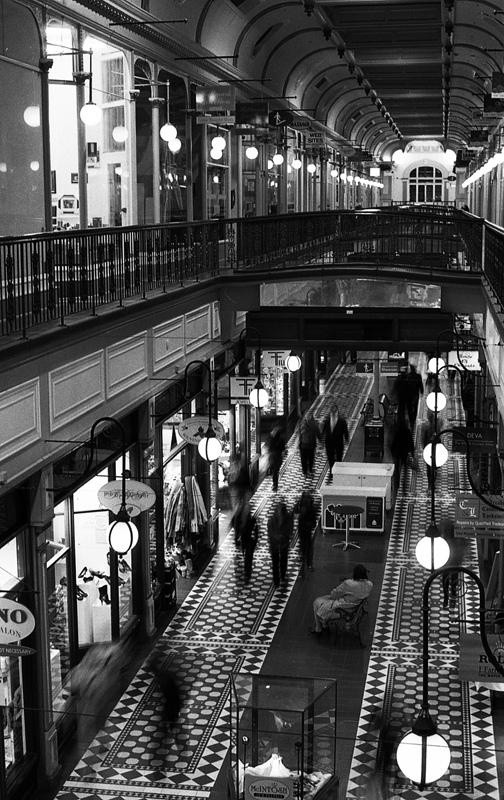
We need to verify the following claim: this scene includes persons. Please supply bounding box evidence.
[232,502,263,583]
[388,366,409,424]
[310,562,374,635]
[421,410,444,490]
[325,402,349,475]
[390,417,416,494]
[298,410,323,477]
[299,489,320,578]
[409,362,425,426]
[266,422,287,494]
[268,503,294,587]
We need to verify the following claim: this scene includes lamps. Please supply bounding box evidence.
[45,43,259,162]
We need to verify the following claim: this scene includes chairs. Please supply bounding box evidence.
[325,597,370,649]
[378,393,397,424]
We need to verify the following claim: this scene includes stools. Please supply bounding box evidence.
[330,505,364,551]
